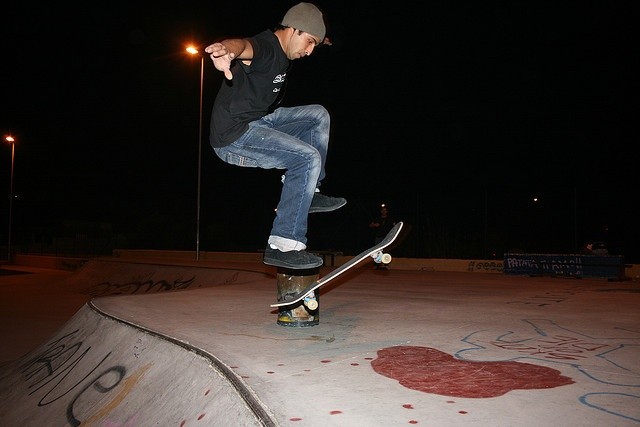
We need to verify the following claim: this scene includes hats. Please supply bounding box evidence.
[281,2,326,45]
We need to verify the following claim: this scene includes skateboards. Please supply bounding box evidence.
[271,222,404,310]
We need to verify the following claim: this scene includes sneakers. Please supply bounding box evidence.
[264,245,324,269]
[308,192,346,213]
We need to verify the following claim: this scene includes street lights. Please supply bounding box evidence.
[5,134,15,258]
[186,45,203,260]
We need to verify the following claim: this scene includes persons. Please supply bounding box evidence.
[204,3,347,269]
